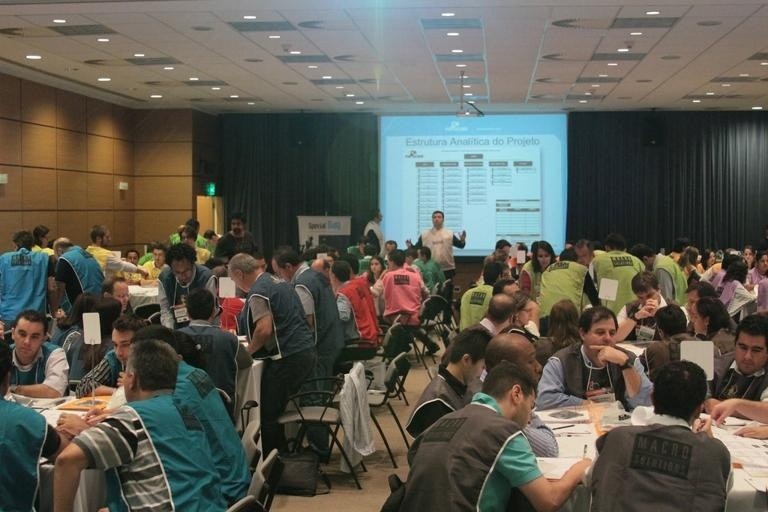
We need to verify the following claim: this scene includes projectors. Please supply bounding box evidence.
[456,112,480,118]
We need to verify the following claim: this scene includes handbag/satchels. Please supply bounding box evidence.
[261,437,322,499]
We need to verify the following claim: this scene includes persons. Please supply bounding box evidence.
[211,211,259,267]
[405,210,468,326]
[362,207,384,253]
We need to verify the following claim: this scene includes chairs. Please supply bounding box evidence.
[1,225,768,512]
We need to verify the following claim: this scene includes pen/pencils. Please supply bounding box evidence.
[552,425,574,430]
[567,435,583,437]
[583,445,587,459]
